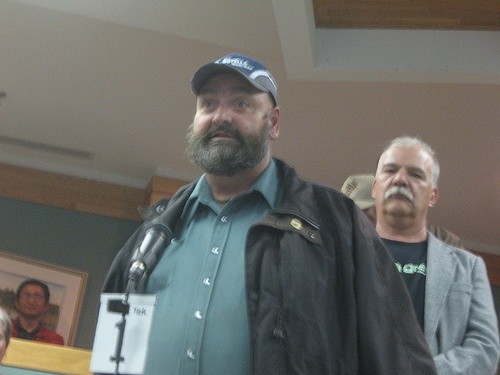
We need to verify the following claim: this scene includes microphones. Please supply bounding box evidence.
[128,219,173,281]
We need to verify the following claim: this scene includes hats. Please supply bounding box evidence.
[190,54,279,107]
[338,175,378,210]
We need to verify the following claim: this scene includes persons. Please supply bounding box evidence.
[0,309,13,364]
[98,54,437,375]
[339,138,500,375]
[9,278,65,346]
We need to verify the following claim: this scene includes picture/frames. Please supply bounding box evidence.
[0,252,89,361]
